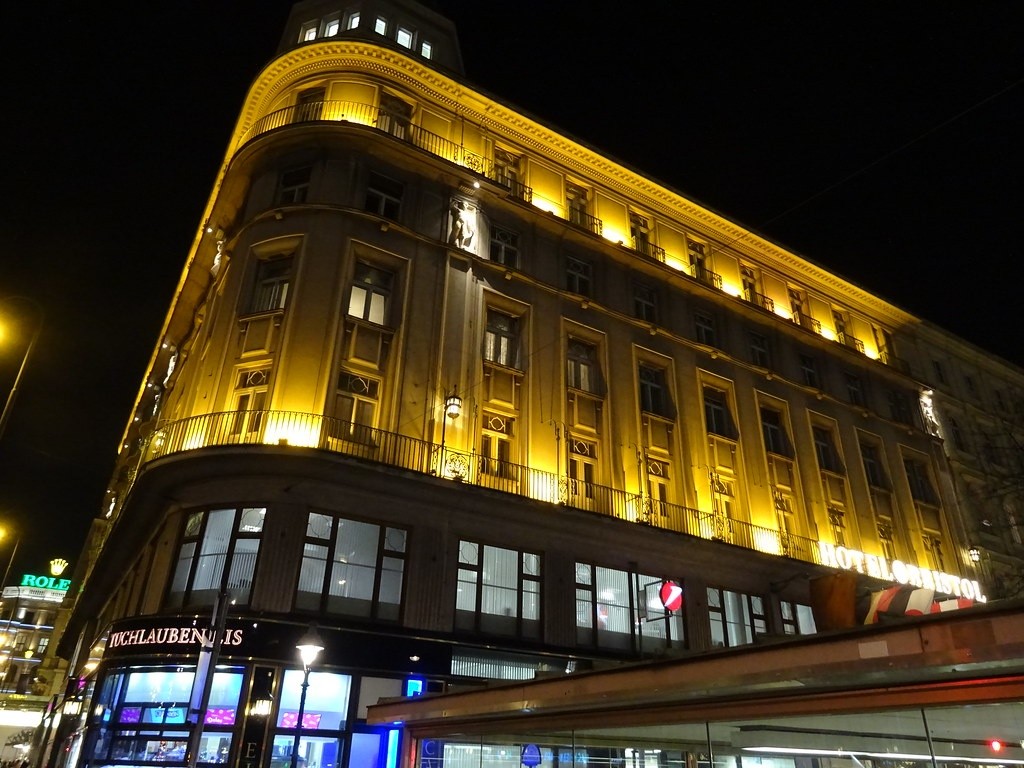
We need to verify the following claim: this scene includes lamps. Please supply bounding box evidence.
[446,385,462,420]
[968,545,980,566]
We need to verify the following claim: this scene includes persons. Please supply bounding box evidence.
[0,758,30,768]
[211,241,223,275]
[32,675,47,695]
[921,390,941,438]
[451,199,476,249]
[164,355,176,388]
[107,498,116,519]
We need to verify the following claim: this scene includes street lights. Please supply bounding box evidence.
[289,624,325,768]
